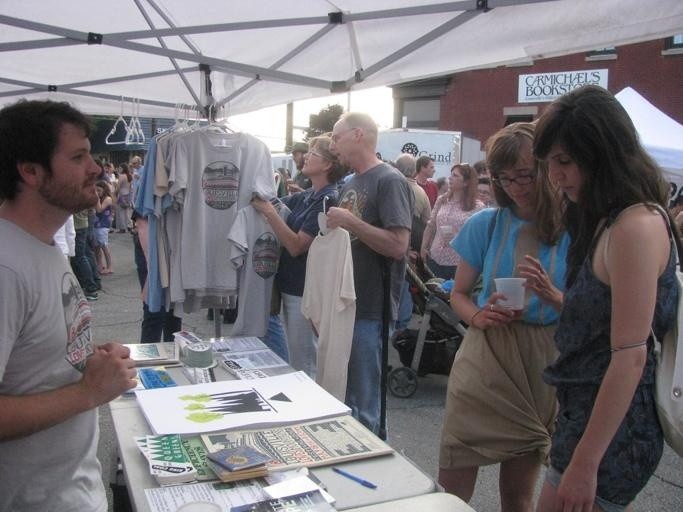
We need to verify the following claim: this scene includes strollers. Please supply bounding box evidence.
[380,259,470,397]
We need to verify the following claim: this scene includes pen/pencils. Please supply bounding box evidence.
[330,465,378,492]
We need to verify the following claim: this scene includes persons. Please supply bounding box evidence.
[94,160,114,185]
[128,155,146,226]
[89,181,114,275]
[476,176,493,203]
[72,207,105,301]
[531,84,682,507]
[285,180,307,195]
[416,156,438,206]
[439,124,567,508]
[277,142,309,190]
[326,111,413,441]
[53,211,76,262]
[394,151,431,305]
[474,163,494,182]
[113,163,132,232]
[420,162,486,278]
[132,211,182,344]
[2,98,136,508]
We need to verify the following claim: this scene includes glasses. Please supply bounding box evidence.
[310,149,329,161]
[330,127,357,145]
[492,173,537,188]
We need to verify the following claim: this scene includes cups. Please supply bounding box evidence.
[494,278,527,311]
[439,225,452,240]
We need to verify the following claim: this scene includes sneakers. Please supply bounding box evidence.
[85,292,98,301]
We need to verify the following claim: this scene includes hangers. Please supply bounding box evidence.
[105,96,135,145]
[318,195,330,235]
[198,103,222,339]
[127,97,146,145]
[268,197,283,208]
[124,97,140,145]
[152,103,239,146]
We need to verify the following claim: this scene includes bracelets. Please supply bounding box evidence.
[470,309,481,325]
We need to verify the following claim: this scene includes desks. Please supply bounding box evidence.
[336,492,478,512]
[109,335,435,512]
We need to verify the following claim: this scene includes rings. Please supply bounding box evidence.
[488,304,498,313]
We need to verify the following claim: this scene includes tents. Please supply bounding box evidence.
[0,1,645,124]
